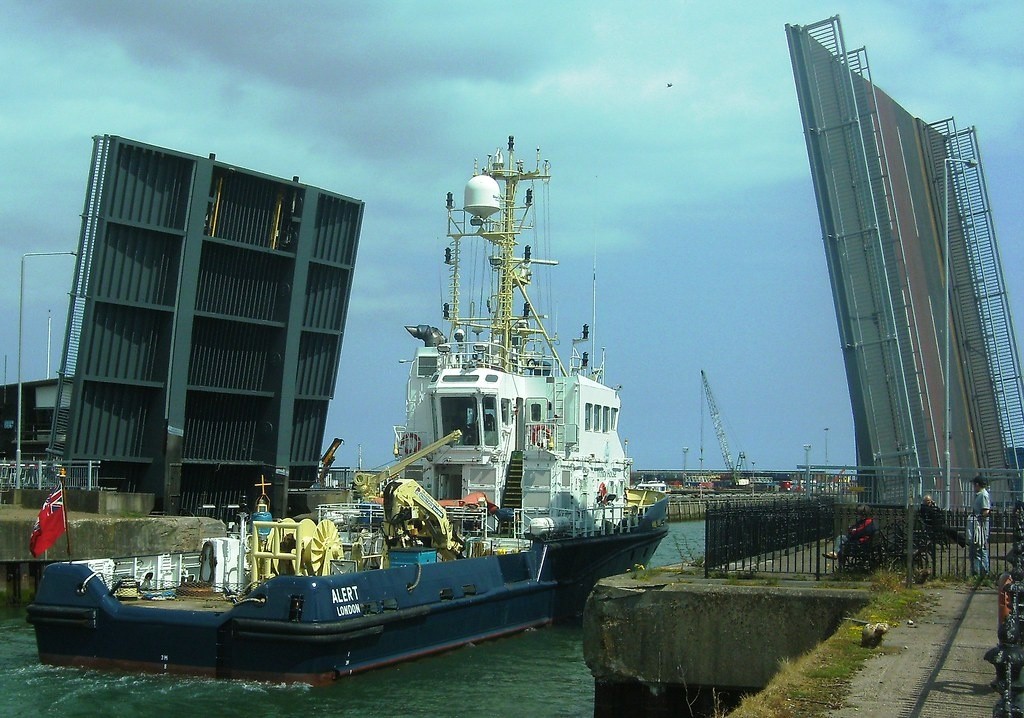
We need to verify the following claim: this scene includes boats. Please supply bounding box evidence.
[25,135,670,687]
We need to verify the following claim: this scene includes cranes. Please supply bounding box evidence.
[699,370,736,475]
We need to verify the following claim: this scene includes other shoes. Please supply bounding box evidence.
[821,551,838,561]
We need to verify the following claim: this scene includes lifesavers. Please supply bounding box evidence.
[400,433,422,454]
[531,425,551,449]
[599,482,607,504]
[997,576,1013,625]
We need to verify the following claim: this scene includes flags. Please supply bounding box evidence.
[29,483,66,559]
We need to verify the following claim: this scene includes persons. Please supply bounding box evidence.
[822,505,876,575]
[921,494,970,549]
[966,475,991,575]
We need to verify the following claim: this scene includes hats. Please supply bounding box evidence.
[969,475,987,485]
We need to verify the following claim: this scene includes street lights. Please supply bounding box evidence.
[46,309,52,379]
[943,158,979,512]
[682,447,689,473]
[751,461,756,498]
[803,443,812,497]
[824,428,829,465]
[15,250,77,488]
